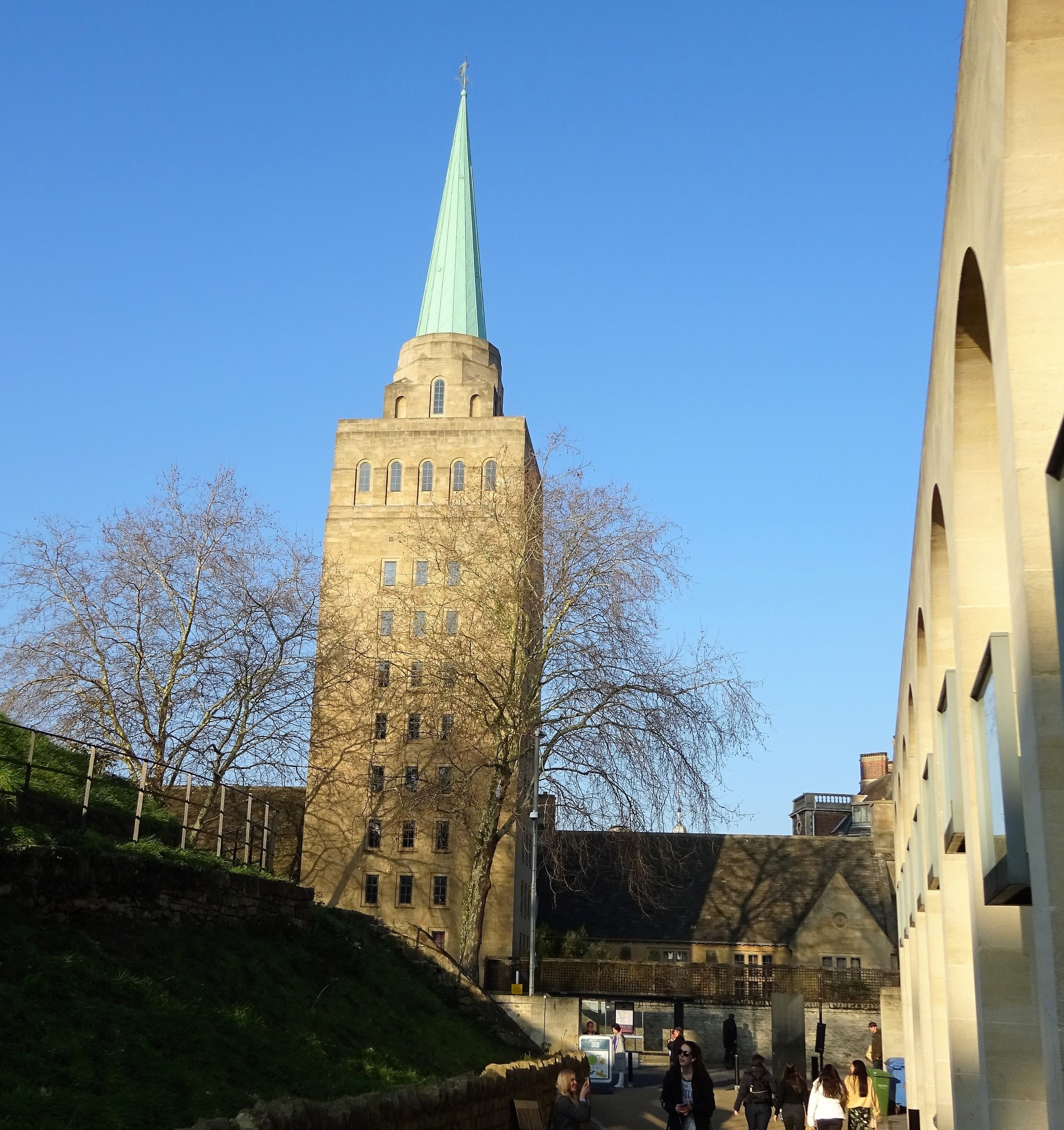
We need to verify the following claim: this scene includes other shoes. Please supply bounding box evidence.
[614,1085,624,1088]
[723,1064,735,1069]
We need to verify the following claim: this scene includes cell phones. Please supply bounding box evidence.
[585,1077,589,1087]
[679,1103,687,1107]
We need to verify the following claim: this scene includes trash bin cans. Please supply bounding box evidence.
[883,1058,906,1115]
[866,1068,892,1116]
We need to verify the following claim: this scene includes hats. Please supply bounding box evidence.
[868,1022,877,1027]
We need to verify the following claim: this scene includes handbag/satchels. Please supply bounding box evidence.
[865,1045,874,1064]
[612,1052,626,1070]
[869,1118,877,1129]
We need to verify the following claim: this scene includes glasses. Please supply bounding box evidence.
[677,1048,691,1057]
[868,1026,872,1028]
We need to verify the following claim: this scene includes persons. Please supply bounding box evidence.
[611,1023,626,1088]
[733,1054,778,1130]
[774,1062,809,1130]
[722,1013,737,1069]
[667,1027,686,1069]
[868,1022,883,1070]
[844,1060,880,1130]
[551,1070,590,1130]
[660,1041,716,1130]
[807,1064,850,1130]
[582,1021,600,1035]
